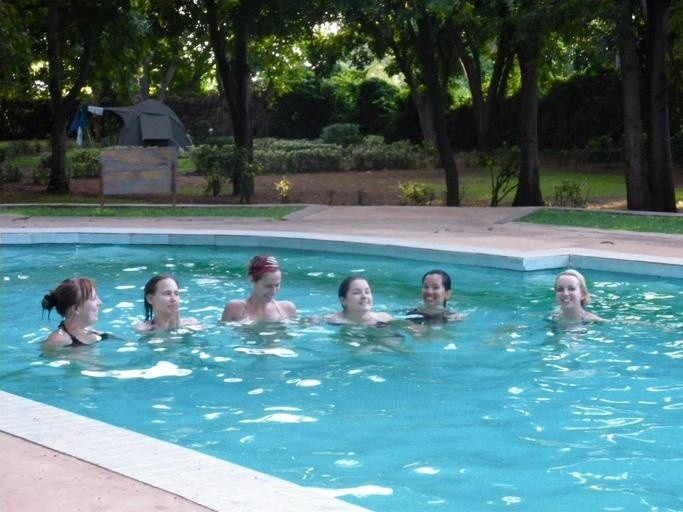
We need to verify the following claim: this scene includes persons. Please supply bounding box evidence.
[548,269,607,322]
[404,269,462,324]
[221,255,296,322]
[41,277,108,347]
[325,276,395,326]
[132,276,199,332]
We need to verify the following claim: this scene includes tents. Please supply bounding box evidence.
[103,98,186,148]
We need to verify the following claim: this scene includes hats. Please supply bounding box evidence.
[249,256,281,276]
